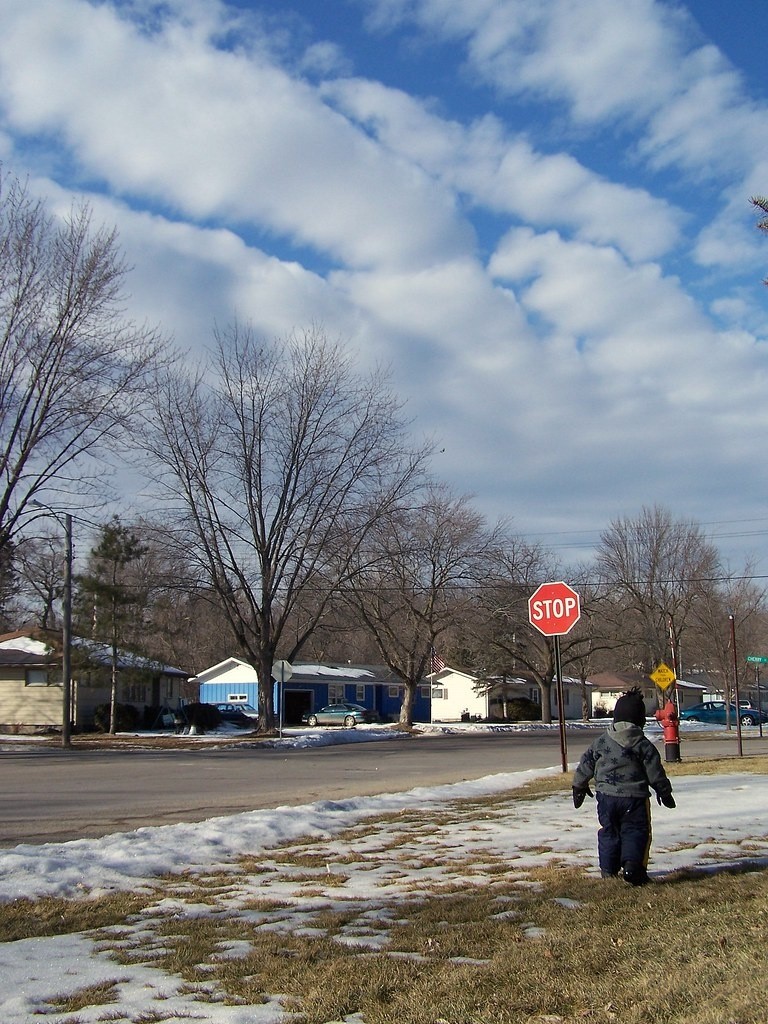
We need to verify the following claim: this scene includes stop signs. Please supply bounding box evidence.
[526,579,581,636]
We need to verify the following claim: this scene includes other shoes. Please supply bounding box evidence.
[623,861,650,886]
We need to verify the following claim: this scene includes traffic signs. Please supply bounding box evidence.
[746,656,768,664]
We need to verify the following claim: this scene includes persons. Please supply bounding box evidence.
[572,686,676,886]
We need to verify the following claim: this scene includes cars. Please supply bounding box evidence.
[212,703,277,724]
[302,705,378,726]
[679,700,766,726]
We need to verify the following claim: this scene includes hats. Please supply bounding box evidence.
[614,686,647,727]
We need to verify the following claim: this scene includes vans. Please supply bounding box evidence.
[734,699,757,710]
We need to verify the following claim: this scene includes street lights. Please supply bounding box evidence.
[27,499,71,748]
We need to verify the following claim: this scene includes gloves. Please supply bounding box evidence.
[657,793,675,808]
[572,786,593,808]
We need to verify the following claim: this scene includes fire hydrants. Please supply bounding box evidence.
[655,700,683,762]
